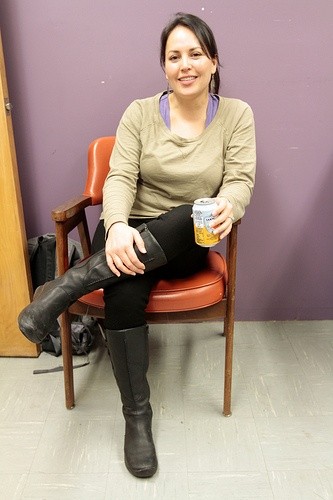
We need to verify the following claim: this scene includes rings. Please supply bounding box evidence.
[228,216,235,221]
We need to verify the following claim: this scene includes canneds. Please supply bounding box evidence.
[192,198,221,247]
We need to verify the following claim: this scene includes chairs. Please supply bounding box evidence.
[52,136,242,418]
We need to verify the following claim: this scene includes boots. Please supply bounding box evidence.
[104,323,158,479]
[17,223,168,345]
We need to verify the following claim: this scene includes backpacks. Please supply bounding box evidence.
[27,232,101,357]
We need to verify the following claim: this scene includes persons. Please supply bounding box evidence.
[18,12,256,479]
